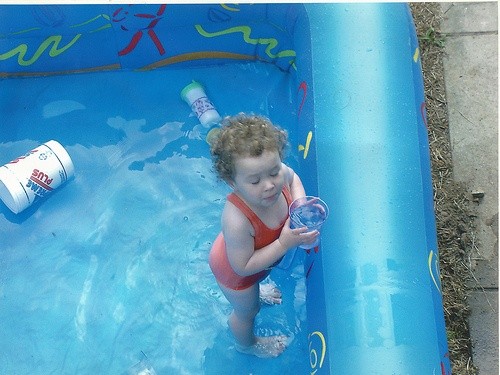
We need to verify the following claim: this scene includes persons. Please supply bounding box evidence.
[207,111,325,346]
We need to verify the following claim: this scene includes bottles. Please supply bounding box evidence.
[179,80,222,128]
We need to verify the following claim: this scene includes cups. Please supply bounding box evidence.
[288,196,329,250]
